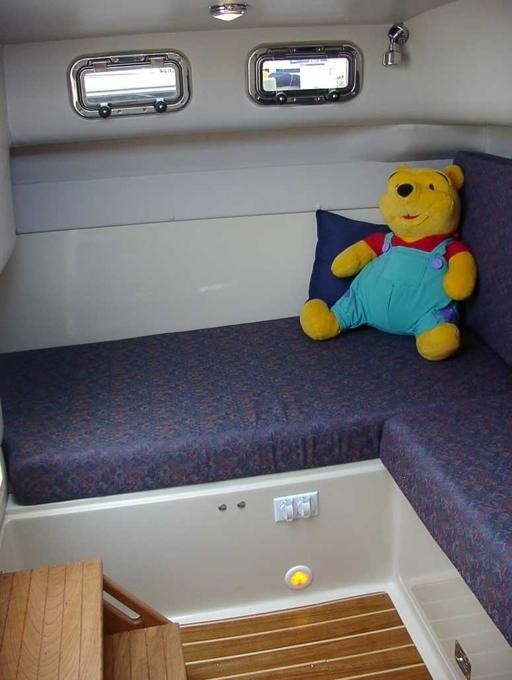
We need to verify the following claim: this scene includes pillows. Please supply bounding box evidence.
[308,209,390,311]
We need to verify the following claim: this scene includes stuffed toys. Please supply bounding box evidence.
[300,160,478,362]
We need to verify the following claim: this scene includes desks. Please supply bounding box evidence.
[0,557,189,680]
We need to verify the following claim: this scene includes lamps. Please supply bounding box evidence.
[210,3,247,21]
[382,22,409,67]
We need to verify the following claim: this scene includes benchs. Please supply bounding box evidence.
[1,150,512,680]
